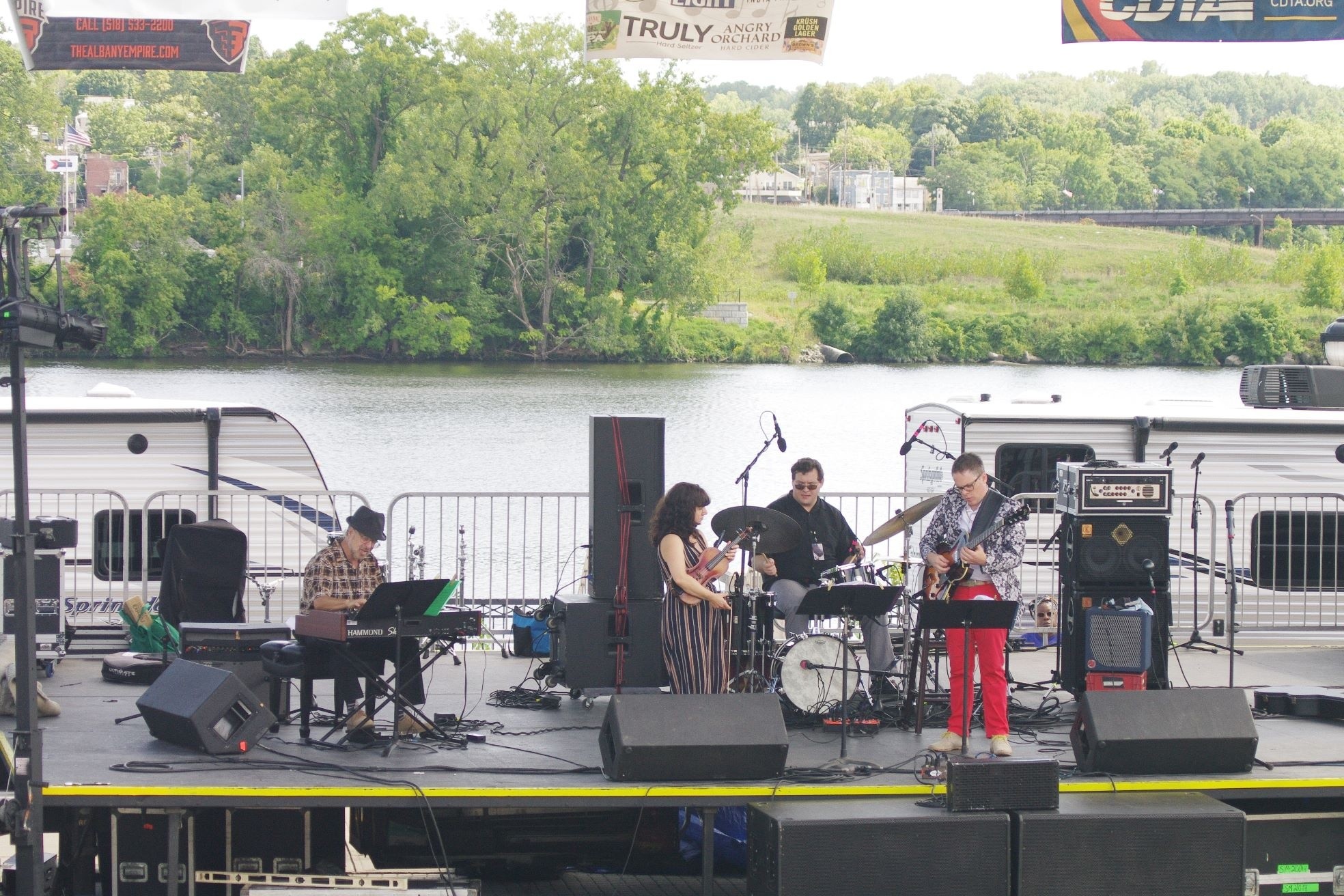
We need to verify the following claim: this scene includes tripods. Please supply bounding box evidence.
[346,579,450,757]
[974,525,1063,701]
[795,516,948,769]
[727,523,796,713]
[286,694,348,724]
[1169,465,1244,655]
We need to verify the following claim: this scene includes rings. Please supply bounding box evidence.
[937,570,939,572]
[964,561,968,564]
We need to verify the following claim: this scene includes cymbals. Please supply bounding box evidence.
[859,494,945,547]
[879,557,924,564]
[709,504,804,556]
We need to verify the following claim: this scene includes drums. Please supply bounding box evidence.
[717,591,778,656]
[819,561,884,618]
[769,632,861,717]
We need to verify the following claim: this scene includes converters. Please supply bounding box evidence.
[467,733,486,743]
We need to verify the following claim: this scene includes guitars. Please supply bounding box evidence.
[666,524,753,607]
[922,504,1032,600]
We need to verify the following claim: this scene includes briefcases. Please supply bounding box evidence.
[0,515,78,549]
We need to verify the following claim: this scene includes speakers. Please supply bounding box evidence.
[747,791,1248,896]
[179,621,292,722]
[588,415,665,602]
[946,757,1060,812]
[1069,688,1258,775]
[136,657,280,755]
[598,694,790,783]
[1060,512,1171,588]
[550,594,670,689]
[1062,585,1169,696]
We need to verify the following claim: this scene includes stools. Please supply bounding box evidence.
[258,637,392,746]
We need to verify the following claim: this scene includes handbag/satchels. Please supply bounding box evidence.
[120,603,180,653]
[508,606,550,657]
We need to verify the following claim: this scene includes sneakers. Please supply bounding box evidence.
[927,731,970,751]
[989,734,1012,756]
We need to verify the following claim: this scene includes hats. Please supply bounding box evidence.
[346,506,388,541]
[1028,596,1058,621]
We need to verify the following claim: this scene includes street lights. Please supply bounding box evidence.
[1251,214,1263,249]
[1246,185,1253,216]
[1153,188,1163,230]
[1015,212,1024,222]
[966,189,975,217]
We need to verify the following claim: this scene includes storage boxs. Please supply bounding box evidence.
[122,596,154,629]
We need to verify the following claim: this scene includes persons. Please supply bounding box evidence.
[747,457,908,692]
[299,506,432,733]
[919,452,1025,756]
[1020,595,1062,648]
[649,482,739,695]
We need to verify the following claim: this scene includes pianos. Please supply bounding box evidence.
[294,606,483,760]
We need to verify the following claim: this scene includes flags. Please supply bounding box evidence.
[66,122,92,146]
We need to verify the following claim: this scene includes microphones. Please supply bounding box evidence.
[1083,448,1094,462]
[1159,442,1178,459]
[900,422,926,455]
[806,662,815,670]
[1142,559,1156,591]
[1225,500,1234,543]
[1191,452,1206,469]
[773,415,786,452]
[849,538,857,556]
[264,677,291,683]
[735,573,740,588]
[875,565,893,573]
[715,529,726,548]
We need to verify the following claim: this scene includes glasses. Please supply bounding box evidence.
[794,480,820,490]
[1037,611,1054,618]
[358,531,380,548]
[953,474,981,494]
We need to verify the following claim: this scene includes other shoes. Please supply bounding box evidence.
[346,713,375,730]
[398,713,437,734]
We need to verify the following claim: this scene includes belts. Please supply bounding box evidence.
[959,580,993,587]
